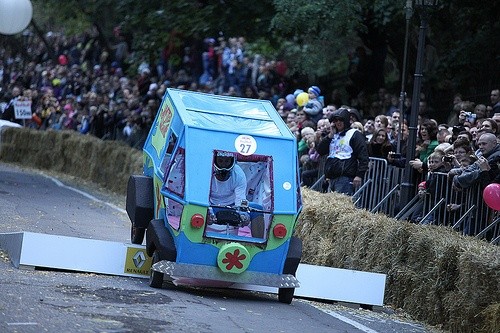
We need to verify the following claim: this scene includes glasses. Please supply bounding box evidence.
[334,118,344,122]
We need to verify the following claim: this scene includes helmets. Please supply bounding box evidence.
[214,150,236,173]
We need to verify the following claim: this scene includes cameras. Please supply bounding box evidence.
[320,127,330,137]
[453,126,463,133]
[464,112,471,118]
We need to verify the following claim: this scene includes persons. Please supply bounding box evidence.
[420,150,449,225]
[208,149,266,239]
[445,156,478,236]
[408,181,427,225]
[452,133,500,241]
[316,109,370,195]
[0,22,500,185]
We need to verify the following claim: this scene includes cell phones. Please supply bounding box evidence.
[391,153,401,159]
[444,157,452,162]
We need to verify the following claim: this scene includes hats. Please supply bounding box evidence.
[64,104,72,110]
[418,180,427,189]
[294,89,304,97]
[308,86,321,96]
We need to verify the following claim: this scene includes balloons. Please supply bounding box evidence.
[0,0,34,36]
[483,182,500,212]
